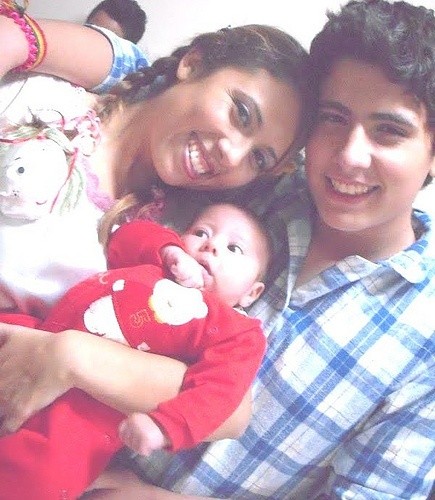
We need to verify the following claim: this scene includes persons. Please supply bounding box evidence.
[0,0,435,500]
[0,25,317,433]
[0,200,274,500]
[85,0,147,45]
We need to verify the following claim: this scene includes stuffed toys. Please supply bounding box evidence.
[1,106,103,231]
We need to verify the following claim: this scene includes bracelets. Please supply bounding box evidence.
[1,0,47,72]
[0,5,39,74]
